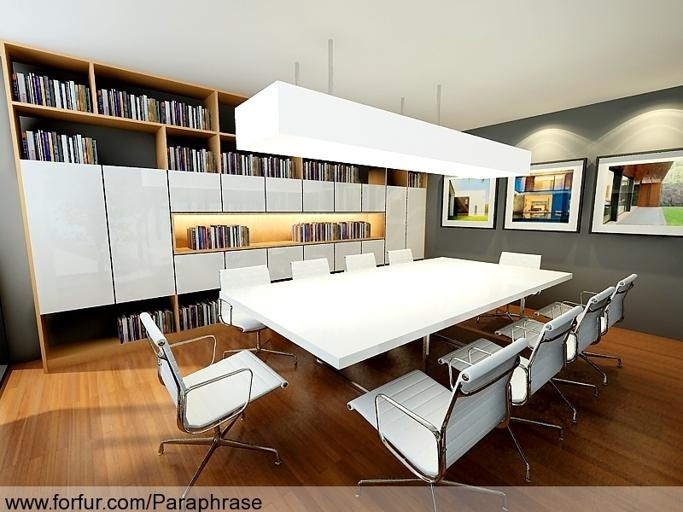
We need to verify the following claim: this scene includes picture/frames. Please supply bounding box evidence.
[440,147,683,238]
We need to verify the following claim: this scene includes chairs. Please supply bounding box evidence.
[137,248,638,512]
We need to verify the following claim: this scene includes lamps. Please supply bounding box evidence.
[233,39,532,183]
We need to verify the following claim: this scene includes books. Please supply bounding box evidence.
[167,145,217,173]
[408,172,421,188]
[304,160,361,183]
[12,71,92,113]
[292,220,371,243]
[21,128,99,165]
[186,224,250,250]
[98,88,213,130]
[116,308,175,344]
[221,151,296,178]
[179,298,219,331]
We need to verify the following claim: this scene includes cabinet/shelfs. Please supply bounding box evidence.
[2,42,429,373]
